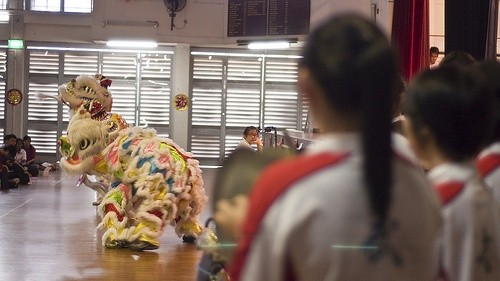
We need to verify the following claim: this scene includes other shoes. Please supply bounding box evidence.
[182,234,197,243]
[13,178,20,183]
[125,241,159,249]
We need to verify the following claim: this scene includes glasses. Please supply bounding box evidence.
[431,54,438,59]
[247,132,258,138]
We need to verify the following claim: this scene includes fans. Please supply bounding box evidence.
[163,0,187,30]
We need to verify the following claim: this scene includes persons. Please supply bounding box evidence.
[235,126,263,152]
[468,61,500,197]
[4,134,39,177]
[227,9,444,280]
[402,67,500,281]
[430,47,439,68]
[0,145,30,190]
[439,50,476,67]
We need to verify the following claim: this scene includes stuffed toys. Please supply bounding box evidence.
[35,74,113,205]
[59,119,217,250]
